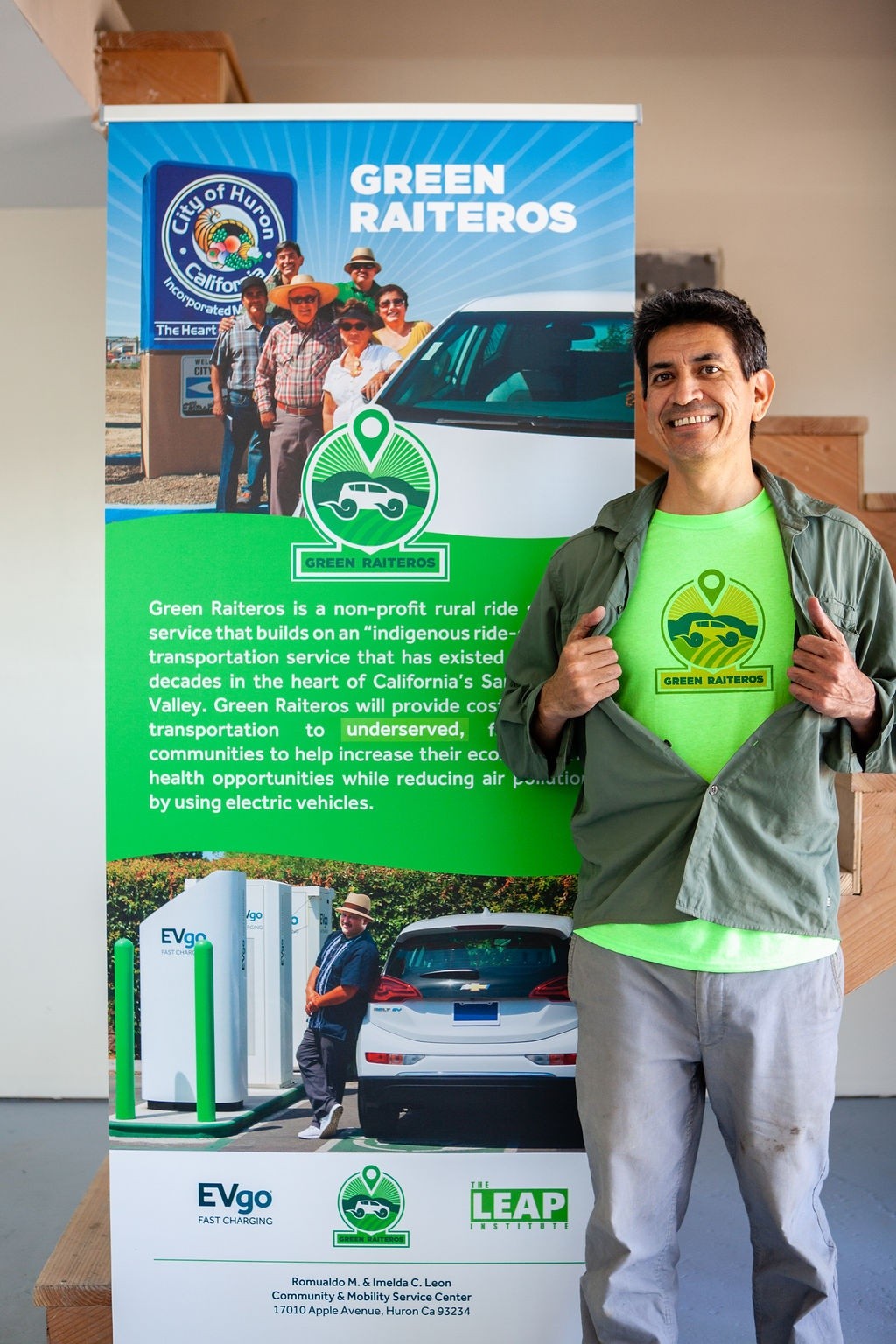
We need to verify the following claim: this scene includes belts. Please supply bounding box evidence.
[276,401,322,416]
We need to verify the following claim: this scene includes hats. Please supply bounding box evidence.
[239,277,267,296]
[267,274,338,310]
[335,892,375,923]
[344,247,381,275]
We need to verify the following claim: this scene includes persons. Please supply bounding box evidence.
[209,240,434,516]
[495,288,896,1344]
[295,891,380,1140]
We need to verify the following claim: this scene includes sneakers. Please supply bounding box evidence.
[320,1103,343,1140]
[297,1126,322,1140]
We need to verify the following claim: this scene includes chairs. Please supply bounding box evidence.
[483,314,601,404]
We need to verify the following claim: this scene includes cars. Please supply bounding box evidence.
[357,913,588,1149]
[286,289,636,538]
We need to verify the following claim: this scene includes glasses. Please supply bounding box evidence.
[350,262,377,269]
[288,294,318,305]
[339,322,368,330]
[379,300,407,308]
